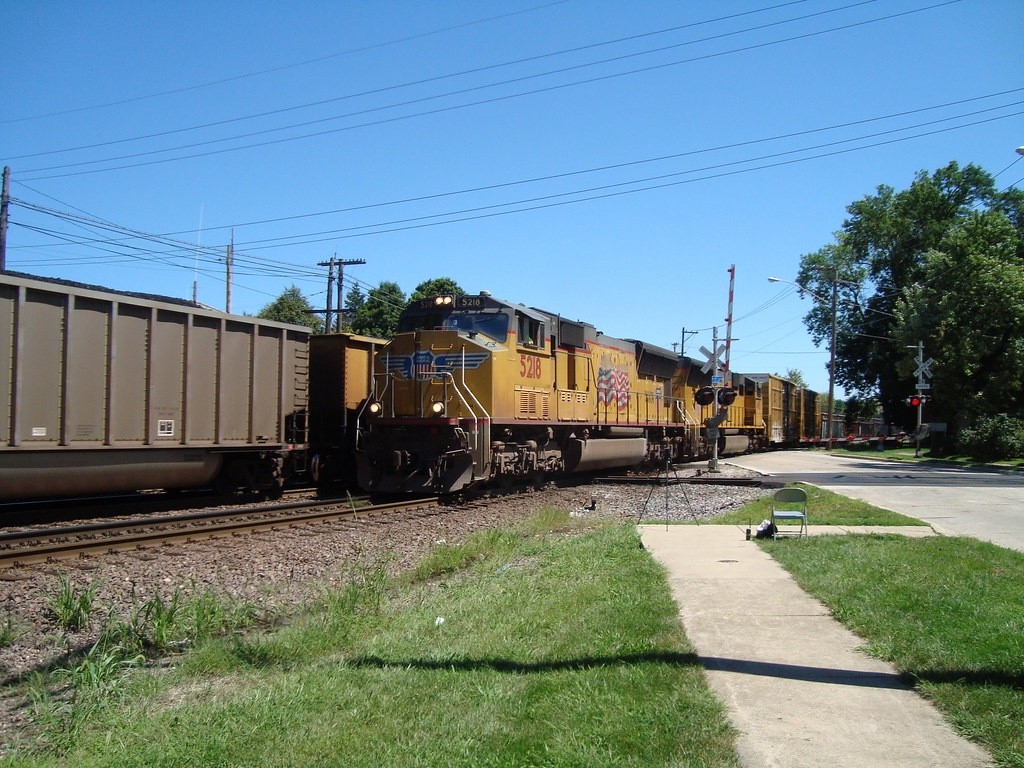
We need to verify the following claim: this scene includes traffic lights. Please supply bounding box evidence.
[718,388,737,406]
[909,395,921,407]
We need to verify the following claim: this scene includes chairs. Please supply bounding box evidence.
[770,488,808,542]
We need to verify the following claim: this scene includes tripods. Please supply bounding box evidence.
[637,448,699,531]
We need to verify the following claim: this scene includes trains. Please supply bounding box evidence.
[353,290,822,493]
[0,274,391,504]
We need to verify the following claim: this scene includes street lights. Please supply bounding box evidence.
[768,277,838,451]
[695,387,715,406]
